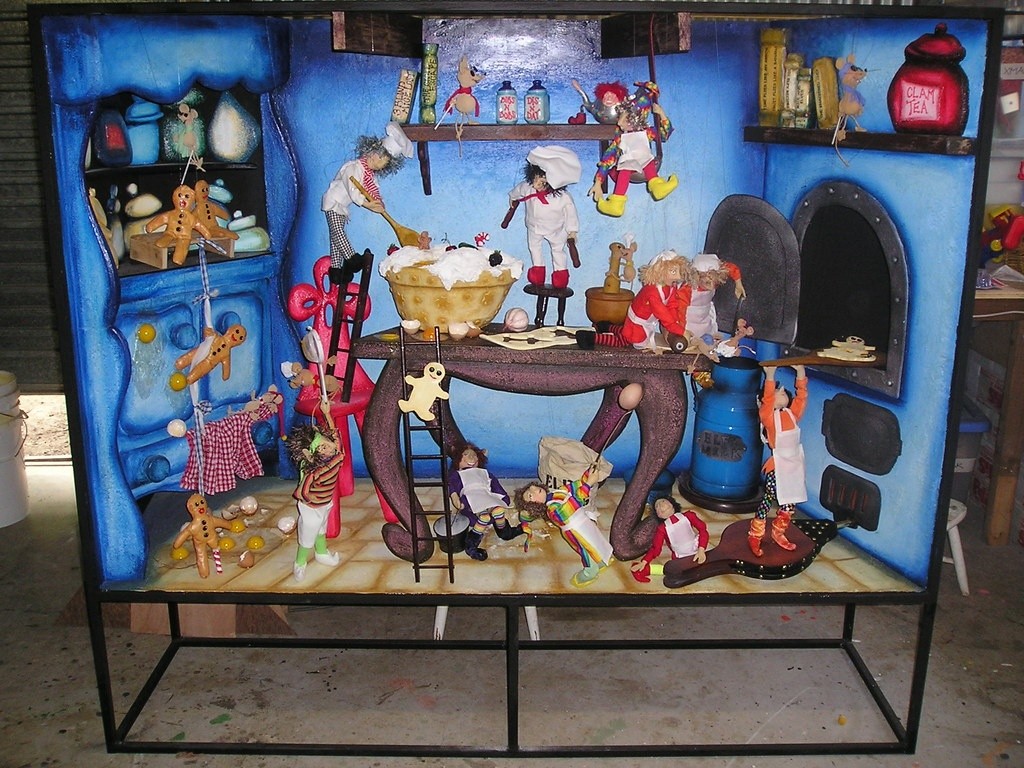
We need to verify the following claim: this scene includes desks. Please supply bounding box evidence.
[972,283,1024,545]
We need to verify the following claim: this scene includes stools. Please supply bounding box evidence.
[942,499,969,595]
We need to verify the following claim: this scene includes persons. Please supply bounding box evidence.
[449,438,525,560]
[514,461,615,585]
[507,145,581,289]
[286,400,346,577]
[575,249,747,354]
[746,365,809,557]
[321,122,414,285]
[630,495,709,583]
[568,80,679,217]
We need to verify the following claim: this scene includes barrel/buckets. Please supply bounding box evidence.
[1,369,31,530]
